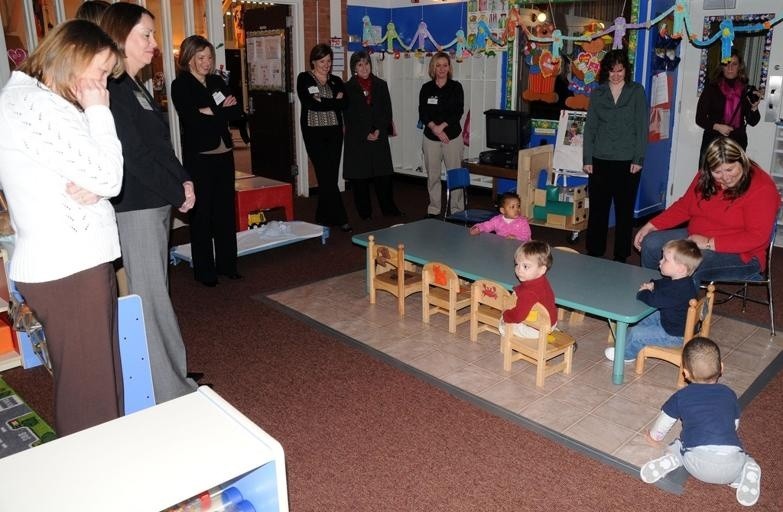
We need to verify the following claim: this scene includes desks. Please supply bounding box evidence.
[234,176,293,231]
[352,219,665,386]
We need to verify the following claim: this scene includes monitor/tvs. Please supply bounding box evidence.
[478,109,531,169]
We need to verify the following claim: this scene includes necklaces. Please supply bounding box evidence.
[314,71,329,84]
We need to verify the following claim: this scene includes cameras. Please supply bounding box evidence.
[746,85,760,103]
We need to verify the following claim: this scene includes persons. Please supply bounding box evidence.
[339,51,407,224]
[604,240,703,364]
[694,48,761,174]
[582,49,649,265]
[634,138,780,297]
[171,35,249,286]
[470,192,532,242]
[418,52,465,224]
[0,0,215,436]
[568,123,578,142]
[640,336,762,506]
[297,43,354,234]
[498,241,559,339]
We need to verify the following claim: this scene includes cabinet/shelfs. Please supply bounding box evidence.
[462,158,517,213]
[0,385,289,510]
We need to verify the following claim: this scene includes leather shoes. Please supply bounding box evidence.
[186,372,204,381]
[198,383,213,389]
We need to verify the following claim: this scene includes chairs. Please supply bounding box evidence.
[443,167,492,227]
[369,235,422,314]
[554,247,580,320]
[635,285,715,388]
[422,263,470,332]
[503,295,576,387]
[469,279,511,354]
[700,217,777,337]
[569,308,629,342]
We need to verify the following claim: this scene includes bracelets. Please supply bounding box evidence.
[706,238,711,250]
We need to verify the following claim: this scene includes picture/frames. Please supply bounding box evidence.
[245,28,285,93]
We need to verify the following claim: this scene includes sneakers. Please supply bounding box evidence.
[360,216,374,221]
[640,454,679,483]
[605,347,636,362]
[448,219,465,226]
[224,272,244,279]
[425,214,444,220]
[736,463,762,507]
[200,277,219,286]
[340,224,352,233]
[388,207,408,218]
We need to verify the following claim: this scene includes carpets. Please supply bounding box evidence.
[249,262,783,496]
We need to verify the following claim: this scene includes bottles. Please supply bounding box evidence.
[168,484,256,512]
